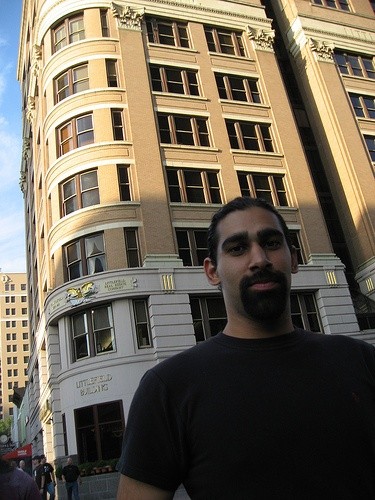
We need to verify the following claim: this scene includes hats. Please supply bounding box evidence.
[31,455,41,460]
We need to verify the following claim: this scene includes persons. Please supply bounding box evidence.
[115,197,375,500]
[19,455,56,500]
[62,458,82,500]
[0,460,41,500]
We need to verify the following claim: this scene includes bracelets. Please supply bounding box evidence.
[41,488,43,489]
